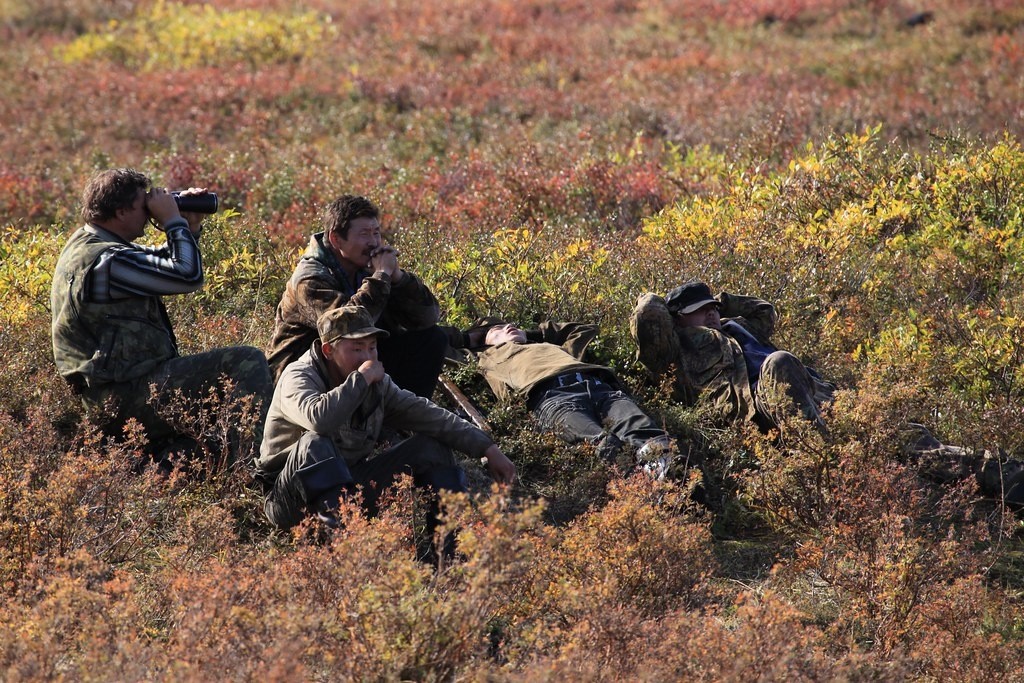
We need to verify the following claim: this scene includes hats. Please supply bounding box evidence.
[315,306,389,343]
[664,282,724,315]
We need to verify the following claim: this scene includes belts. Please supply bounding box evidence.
[527,372,602,396]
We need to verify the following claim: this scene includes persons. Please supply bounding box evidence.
[264,195,450,401]
[49,168,275,466]
[255,304,518,563]
[441,316,707,507]
[631,281,1024,509]
